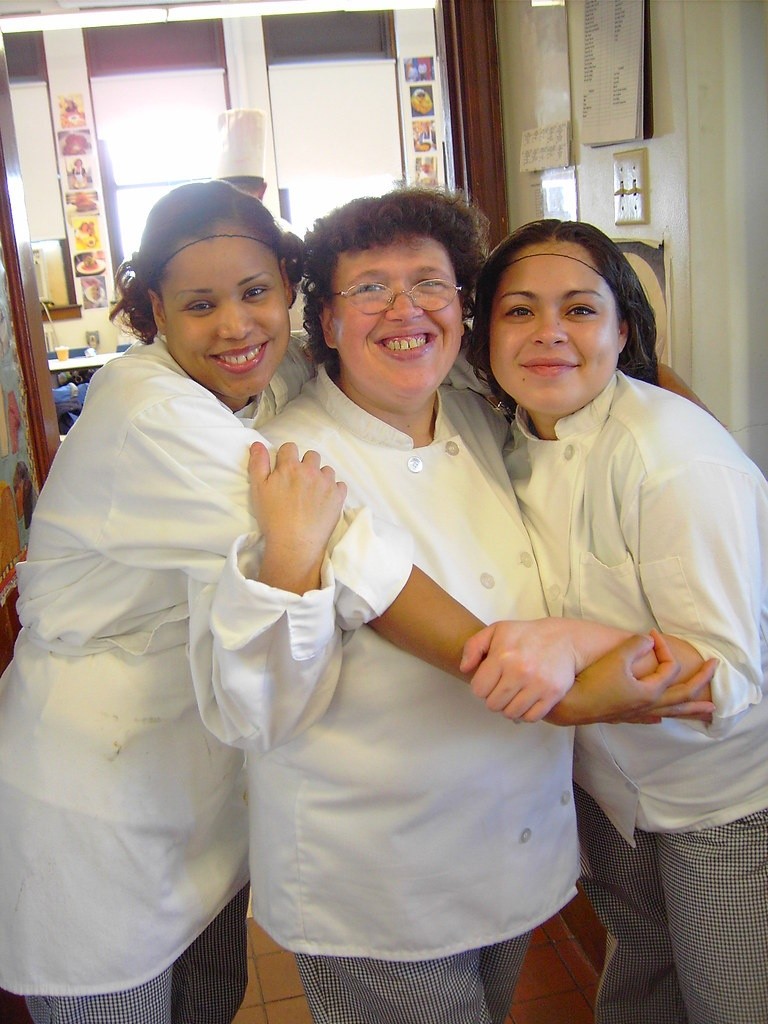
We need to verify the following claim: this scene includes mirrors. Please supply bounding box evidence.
[30,240,74,309]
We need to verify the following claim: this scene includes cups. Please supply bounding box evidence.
[55,346,68,361]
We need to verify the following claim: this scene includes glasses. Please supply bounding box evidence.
[324,278,462,315]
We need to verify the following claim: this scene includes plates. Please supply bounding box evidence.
[76,259,106,275]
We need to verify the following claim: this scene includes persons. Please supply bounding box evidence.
[217,108,307,331]
[0,184,718,1024]
[466,218,768,1024]
[192,190,579,1024]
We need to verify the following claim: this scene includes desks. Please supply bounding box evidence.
[48,351,124,371]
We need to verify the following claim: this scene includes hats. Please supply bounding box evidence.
[213,109,263,180]
